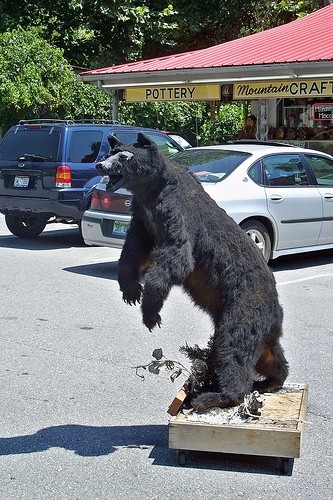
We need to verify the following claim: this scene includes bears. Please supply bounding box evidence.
[93,130,289,415]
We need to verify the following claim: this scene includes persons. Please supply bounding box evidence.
[244,114,257,139]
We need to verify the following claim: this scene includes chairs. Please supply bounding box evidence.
[272,167,295,187]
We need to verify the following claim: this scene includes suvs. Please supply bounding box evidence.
[0,118,185,242]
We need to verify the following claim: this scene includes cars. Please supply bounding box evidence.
[82,143,333,266]
[161,130,191,150]
